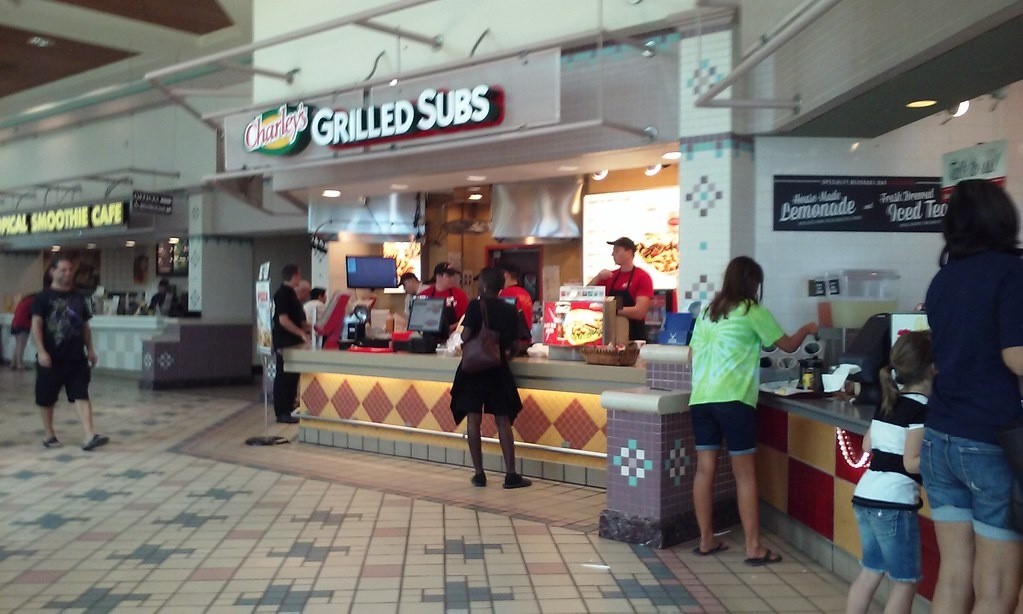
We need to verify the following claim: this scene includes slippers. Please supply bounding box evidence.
[43,436,63,447]
[503,476,532,488]
[472,475,486,486]
[693,541,729,555]
[745,548,782,566]
[82,434,109,450]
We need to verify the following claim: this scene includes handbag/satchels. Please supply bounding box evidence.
[461,300,501,370]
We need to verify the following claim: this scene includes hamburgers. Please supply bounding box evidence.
[563,309,604,345]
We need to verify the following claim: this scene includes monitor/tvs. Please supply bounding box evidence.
[408,298,446,333]
[890,313,931,347]
[346,256,398,290]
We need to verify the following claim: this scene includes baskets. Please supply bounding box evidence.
[582,346,639,366]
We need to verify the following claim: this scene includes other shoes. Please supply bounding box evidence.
[278,412,299,423]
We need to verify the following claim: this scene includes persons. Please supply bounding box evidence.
[686,256,818,567]
[844,330,941,614]
[449,267,532,489]
[586,237,653,343]
[28,255,110,450]
[918,179,1023,614]
[10,293,36,371]
[417,260,538,354]
[272,263,328,424]
[398,272,431,336]
[149,279,188,311]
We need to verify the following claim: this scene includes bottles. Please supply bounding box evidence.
[386,312,394,334]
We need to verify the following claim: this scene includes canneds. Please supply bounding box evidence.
[555,302,571,340]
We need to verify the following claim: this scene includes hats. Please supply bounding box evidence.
[435,262,456,275]
[607,237,636,252]
[396,273,415,287]
